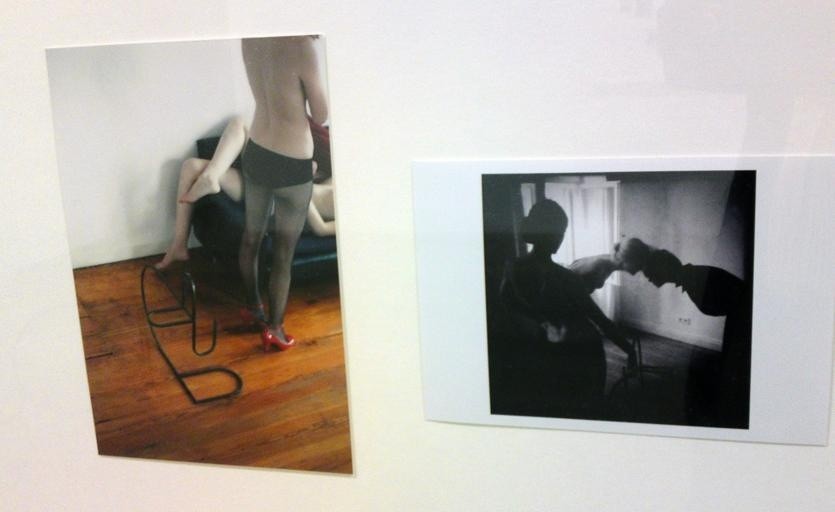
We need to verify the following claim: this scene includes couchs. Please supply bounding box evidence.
[188,127,338,304]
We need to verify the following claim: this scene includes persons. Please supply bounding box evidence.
[572,238,649,399]
[642,249,752,428]
[495,200,638,417]
[153,115,336,271]
[240,35,327,352]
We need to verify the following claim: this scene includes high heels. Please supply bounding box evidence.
[262,325,295,351]
[240,304,265,326]
